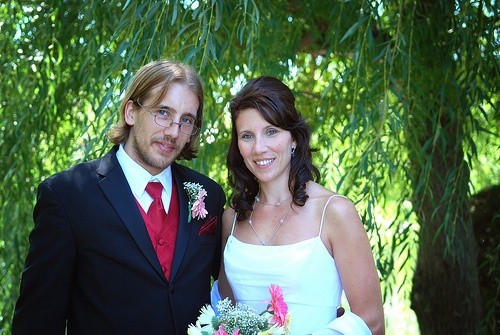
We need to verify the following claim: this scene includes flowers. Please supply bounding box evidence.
[187,276,292,335]
[183,182,216,222]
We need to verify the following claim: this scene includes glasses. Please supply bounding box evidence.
[137,99,199,136]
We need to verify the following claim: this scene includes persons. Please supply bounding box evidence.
[217,77,384,335]
[12,62,225,334]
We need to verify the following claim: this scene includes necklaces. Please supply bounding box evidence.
[248,194,294,245]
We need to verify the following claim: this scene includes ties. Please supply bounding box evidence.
[145,181,167,229]
